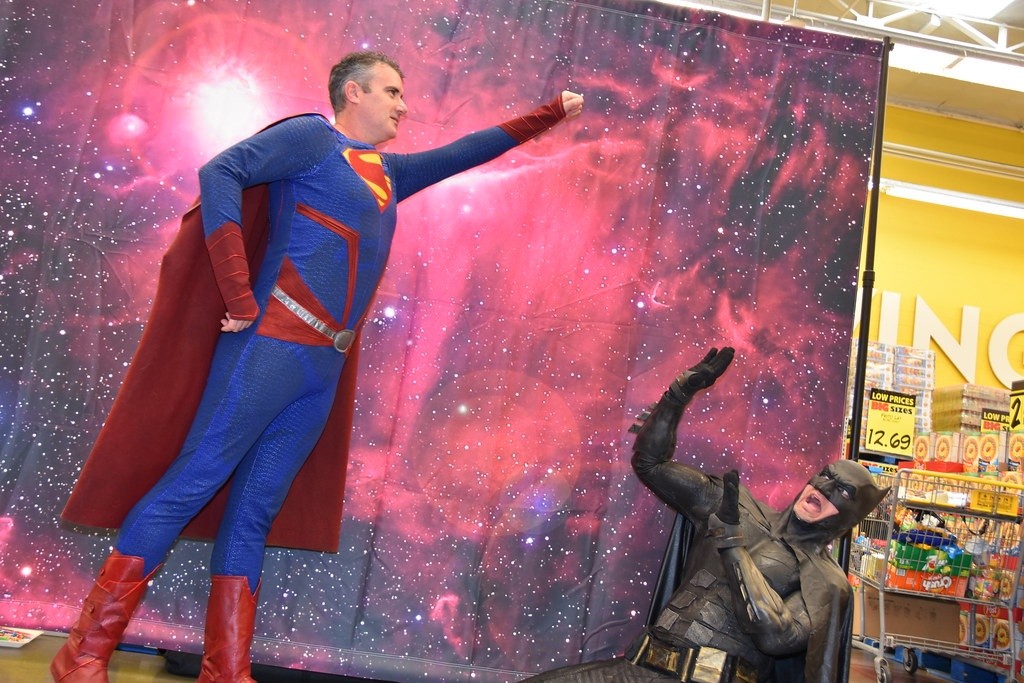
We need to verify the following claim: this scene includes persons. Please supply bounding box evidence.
[49,50,585,683]
[513,343,891,682]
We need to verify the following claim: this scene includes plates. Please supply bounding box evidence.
[0,626,45,648]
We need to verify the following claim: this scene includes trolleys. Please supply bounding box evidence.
[847,468,1024,683]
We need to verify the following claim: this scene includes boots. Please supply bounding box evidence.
[51,549,163,682]
[197,577,264,683]
